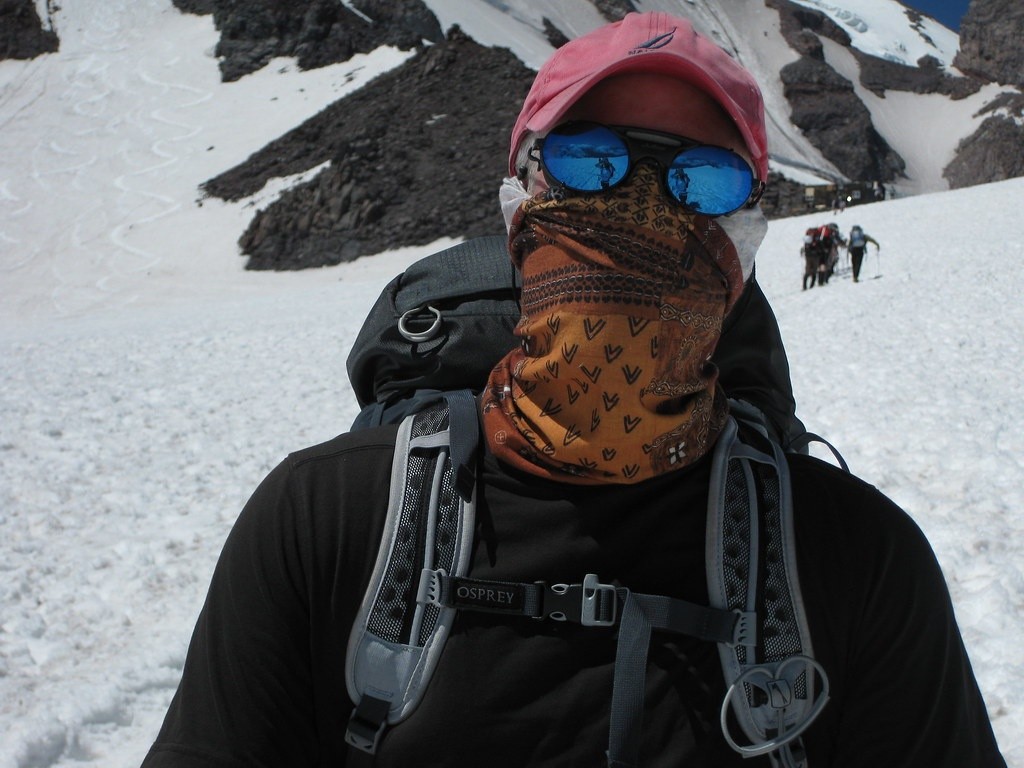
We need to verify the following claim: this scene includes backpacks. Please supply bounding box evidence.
[819,226,831,243]
[851,231,866,248]
[347,233,830,768]
[805,228,818,256]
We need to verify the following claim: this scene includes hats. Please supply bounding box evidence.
[507,11,766,190]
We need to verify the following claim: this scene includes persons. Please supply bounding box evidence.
[803,223,879,291]
[595,156,615,189]
[140,9,1007,768]
[670,166,690,204]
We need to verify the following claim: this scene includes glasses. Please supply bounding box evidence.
[517,120,766,217]
